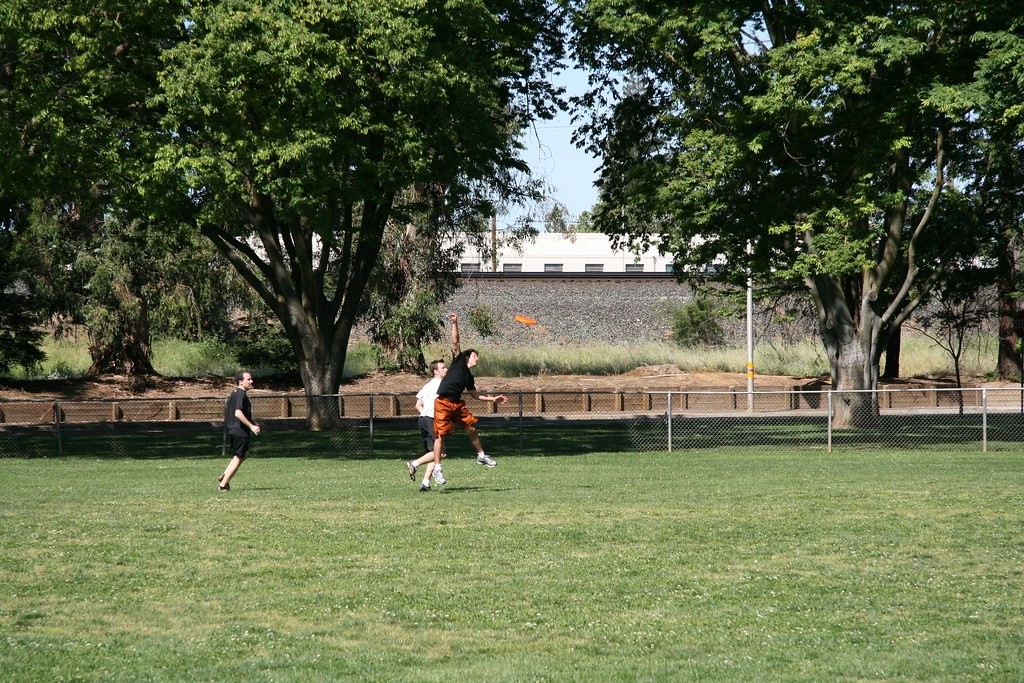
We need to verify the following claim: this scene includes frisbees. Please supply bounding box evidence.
[515,316,535,325]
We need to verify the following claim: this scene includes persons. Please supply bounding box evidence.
[216,370,261,493]
[433,313,497,485]
[405,358,449,493]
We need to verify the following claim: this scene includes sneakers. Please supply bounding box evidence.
[406,462,416,481]
[218,474,230,492]
[419,484,431,493]
[476,455,497,468]
[432,468,446,484]
